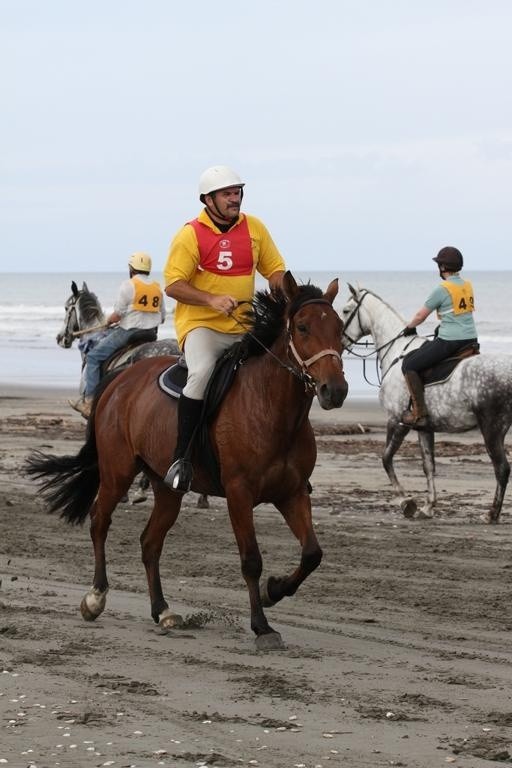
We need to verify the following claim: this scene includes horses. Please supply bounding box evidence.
[53,280,211,511]
[23,270,349,654]
[336,280,510,524]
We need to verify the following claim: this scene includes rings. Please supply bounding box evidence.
[223,302,228,308]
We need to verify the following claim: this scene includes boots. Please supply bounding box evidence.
[168,393,206,493]
[402,372,427,427]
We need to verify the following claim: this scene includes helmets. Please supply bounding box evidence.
[198,166,246,203]
[128,253,152,273]
[433,247,463,266]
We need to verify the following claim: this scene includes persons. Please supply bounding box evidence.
[161,164,288,494]
[397,246,477,429]
[67,250,168,422]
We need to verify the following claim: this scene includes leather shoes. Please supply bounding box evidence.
[68,399,92,419]
